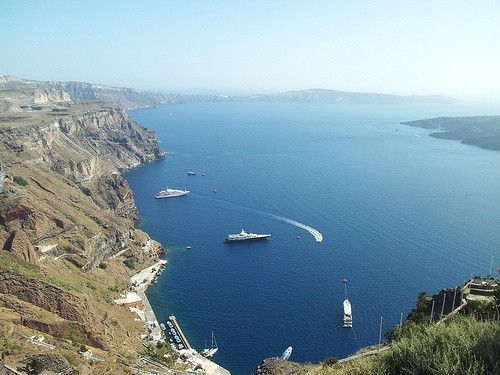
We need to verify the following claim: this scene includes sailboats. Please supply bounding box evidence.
[200,331,218,358]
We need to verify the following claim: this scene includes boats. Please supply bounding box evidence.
[343,299,353,327]
[224,229,270,242]
[284,346,292,353]
[160,321,184,349]
[155,186,190,199]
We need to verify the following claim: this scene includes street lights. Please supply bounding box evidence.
[378,256,500,348]
